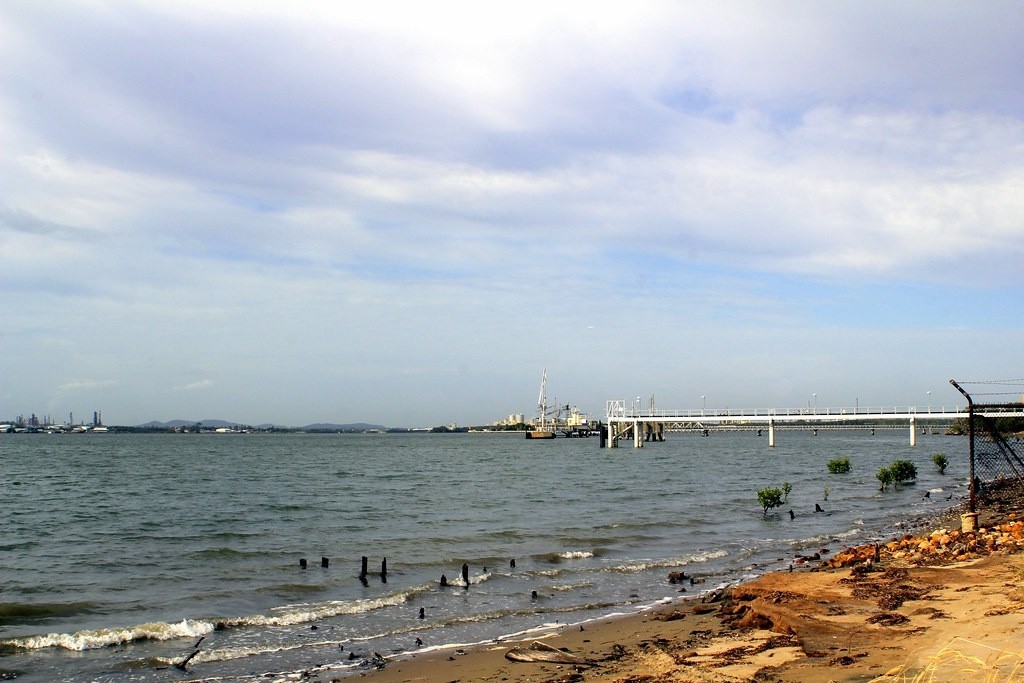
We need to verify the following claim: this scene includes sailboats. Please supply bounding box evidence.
[524,367,558,440]
